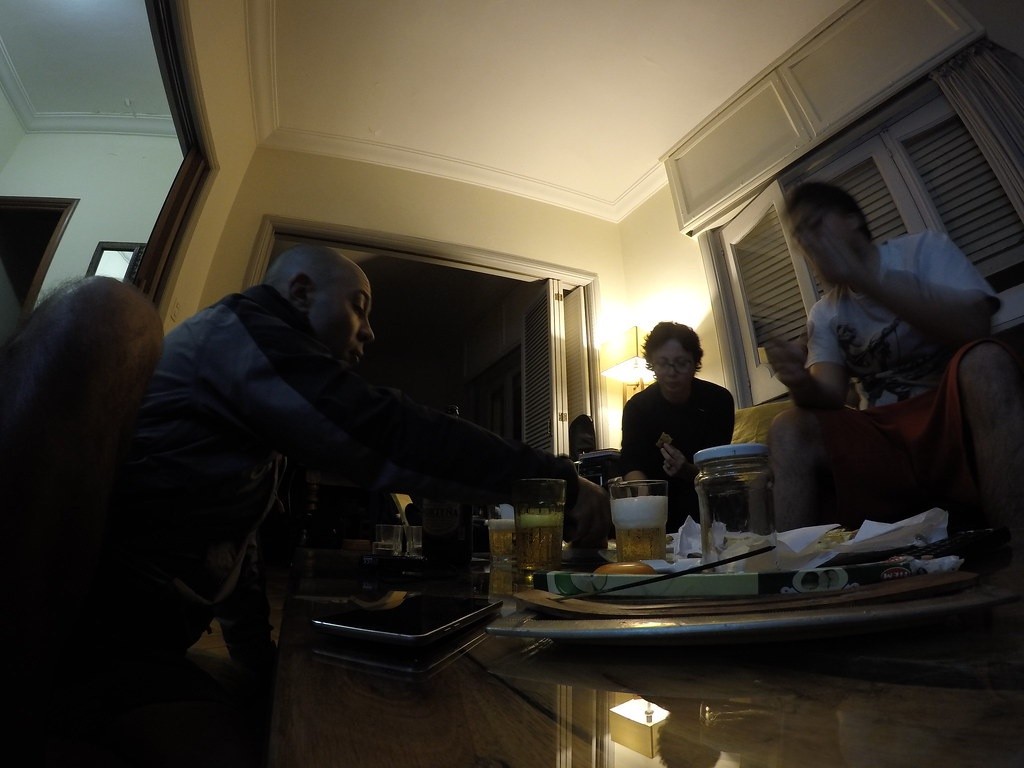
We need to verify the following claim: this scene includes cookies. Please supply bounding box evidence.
[656,432,673,448]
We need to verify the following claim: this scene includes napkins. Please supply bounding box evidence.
[635,507,949,574]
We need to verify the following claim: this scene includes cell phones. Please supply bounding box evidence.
[309,593,505,647]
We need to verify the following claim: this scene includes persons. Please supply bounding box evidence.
[70,243,609,768]
[767,179,1024,534]
[640,696,720,768]
[569,323,735,531]
[0,279,163,768]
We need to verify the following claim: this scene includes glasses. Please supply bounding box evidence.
[651,359,696,374]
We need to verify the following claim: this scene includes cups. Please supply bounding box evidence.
[488,563,511,608]
[515,478,567,570]
[471,505,490,561]
[609,479,668,561]
[404,525,425,557]
[489,506,515,562]
[375,525,404,557]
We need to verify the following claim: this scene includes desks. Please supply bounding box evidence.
[266,582,1024,768]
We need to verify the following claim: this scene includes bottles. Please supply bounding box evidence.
[421,405,463,539]
[693,444,776,573]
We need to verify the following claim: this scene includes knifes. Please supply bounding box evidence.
[627,589,858,610]
